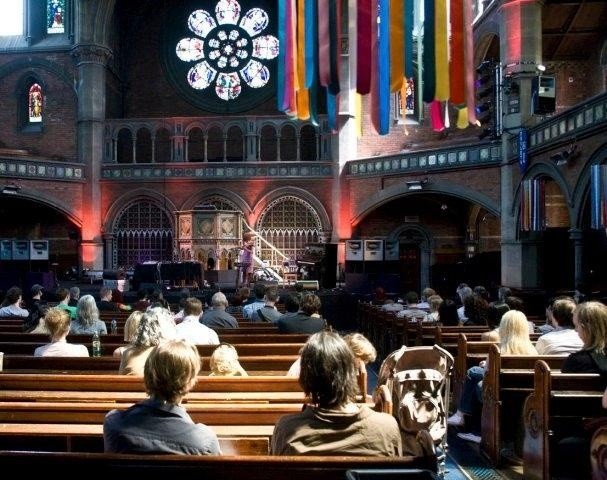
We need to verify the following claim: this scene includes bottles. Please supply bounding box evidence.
[92,330,101,357]
[110,318,118,335]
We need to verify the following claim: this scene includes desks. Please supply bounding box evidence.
[131,264,204,290]
[204,270,236,283]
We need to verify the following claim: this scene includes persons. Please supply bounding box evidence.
[531,297,586,355]
[118,308,179,376]
[1,285,29,316]
[235,231,256,287]
[559,300,607,384]
[25,273,607,345]
[208,342,250,377]
[100,338,227,456]
[33,307,90,356]
[285,329,378,380]
[445,309,542,445]
[268,329,405,459]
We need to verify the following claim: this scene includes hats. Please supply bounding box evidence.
[30,284,44,294]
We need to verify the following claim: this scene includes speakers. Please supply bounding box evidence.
[294,279,318,291]
[531,74,558,114]
[345,236,399,261]
[345,273,377,293]
[204,270,236,291]
[0,240,49,260]
[131,259,204,292]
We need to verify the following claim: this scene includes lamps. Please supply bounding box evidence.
[406,178,427,191]
[3,183,21,195]
[549,145,577,167]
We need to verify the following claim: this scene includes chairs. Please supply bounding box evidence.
[282,262,297,289]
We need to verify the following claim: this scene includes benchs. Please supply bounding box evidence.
[344,299,607,480]
[1,303,439,478]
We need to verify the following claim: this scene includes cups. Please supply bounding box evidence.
[0,351,4,371]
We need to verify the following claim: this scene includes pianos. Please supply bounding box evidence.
[296,242,337,288]
